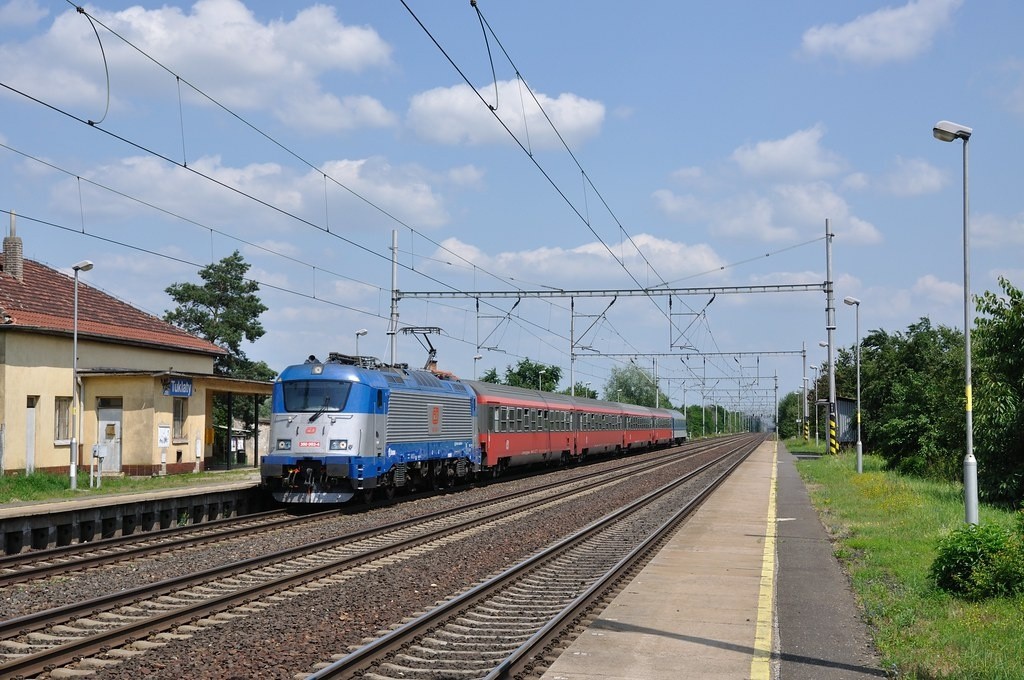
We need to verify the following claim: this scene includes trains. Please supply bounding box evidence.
[261,362,686,503]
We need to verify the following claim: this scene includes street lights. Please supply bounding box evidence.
[617,389,622,403]
[586,382,592,398]
[794,341,829,446]
[69,260,93,491]
[844,296,862,473]
[473,355,483,381]
[355,328,368,356]
[932,120,978,524]
[539,371,546,391]
